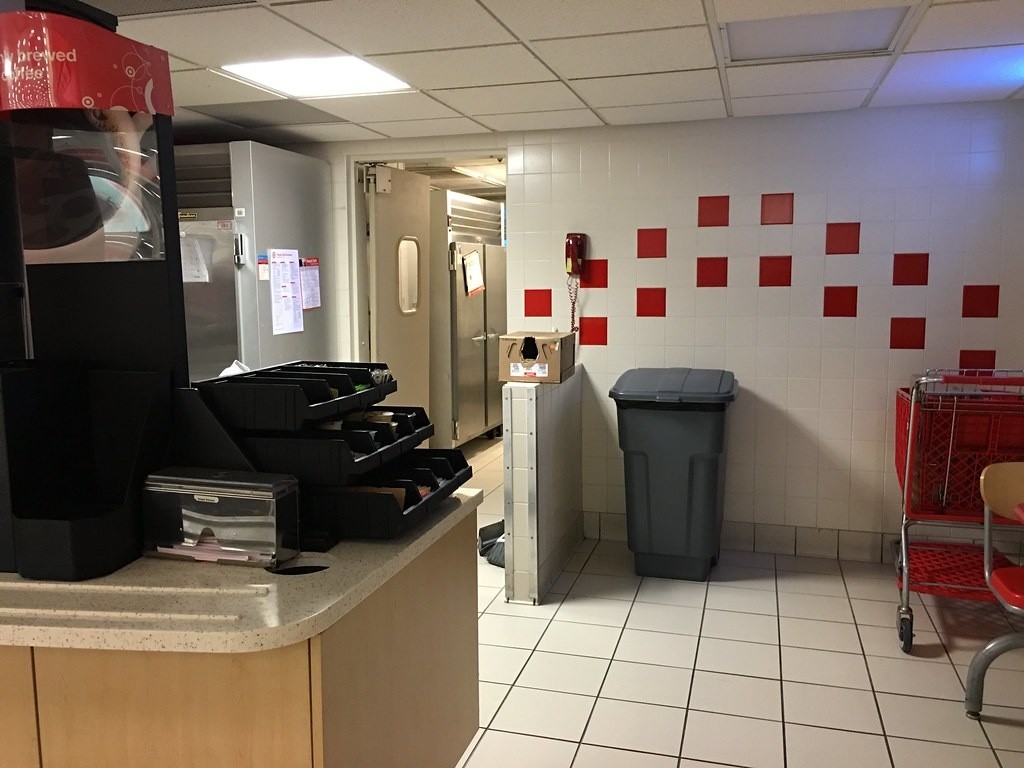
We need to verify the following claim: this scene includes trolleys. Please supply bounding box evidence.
[892,370,1024,652]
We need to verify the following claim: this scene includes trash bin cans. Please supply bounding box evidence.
[607,366,741,584]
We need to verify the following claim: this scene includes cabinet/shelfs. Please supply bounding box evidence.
[0,507,479,768]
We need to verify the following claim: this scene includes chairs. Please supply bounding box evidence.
[964,462,1024,721]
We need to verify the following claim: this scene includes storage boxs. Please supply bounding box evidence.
[498,331,576,385]
